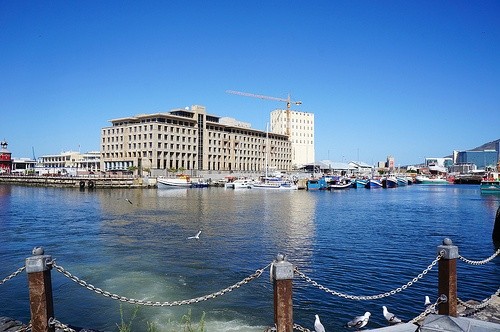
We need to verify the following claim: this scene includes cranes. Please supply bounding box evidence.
[225,90,302,136]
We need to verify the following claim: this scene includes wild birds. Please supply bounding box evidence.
[424,295,433,307]
[314,314,325,332]
[342,311,372,329]
[188,231,201,239]
[381,306,402,325]
[125,198,133,205]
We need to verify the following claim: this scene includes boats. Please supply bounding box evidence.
[324,174,451,190]
[225,176,252,189]
[156,178,194,188]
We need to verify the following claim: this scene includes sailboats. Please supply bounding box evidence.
[251,122,298,191]
